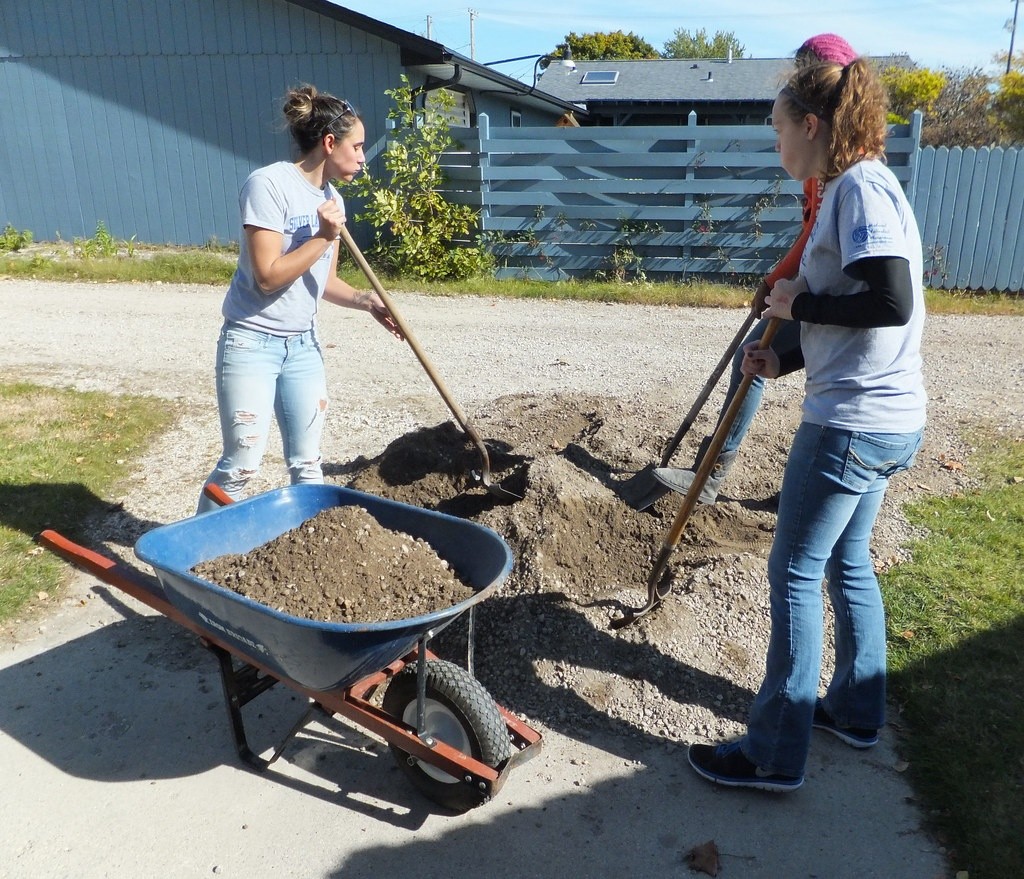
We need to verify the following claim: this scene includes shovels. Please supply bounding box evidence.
[608,317,788,628]
[338,224,523,504]
[622,307,760,512]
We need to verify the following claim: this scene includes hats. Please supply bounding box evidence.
[794,33,857,70]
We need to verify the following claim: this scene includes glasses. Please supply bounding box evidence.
[316,99,357,137]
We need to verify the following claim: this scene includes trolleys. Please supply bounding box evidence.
[42,479,547,816]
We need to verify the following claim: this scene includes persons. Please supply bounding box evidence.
[652,32,863,508]
[686,56,932,801]
[194,84,404,521]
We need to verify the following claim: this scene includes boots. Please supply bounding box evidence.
[651,436,738,506]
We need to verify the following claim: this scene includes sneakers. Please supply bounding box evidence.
[688,741,804,793]
[812,696,878,748]
